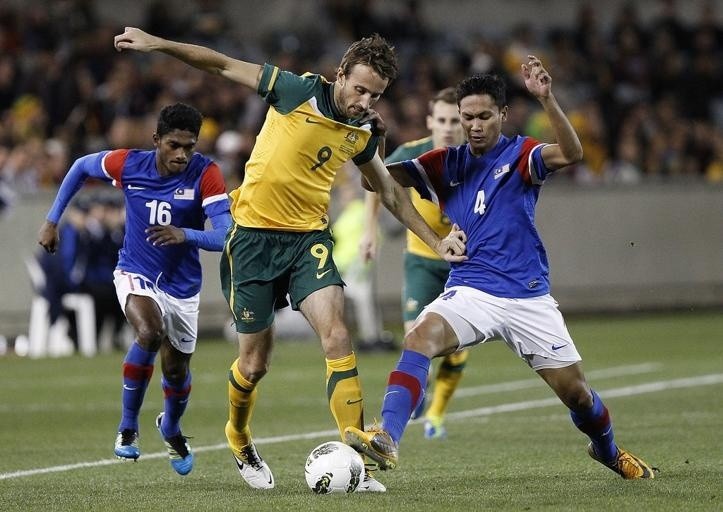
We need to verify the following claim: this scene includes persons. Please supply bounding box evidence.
[327,182,398,348]
[113,26,469,490]
[21,187,135,360]
[2,0,723,213]
[341,54,655,480]
[358,85,469,442]
[40,103,234,476]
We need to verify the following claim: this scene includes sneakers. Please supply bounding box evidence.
[357,472,386,493]
[156,412,192,475]
[410,364,432,418]
[114,426,140,458]
[343,427,398,469]
[588,441,654,479]
[227,438,274,489]
[423,416,446,441]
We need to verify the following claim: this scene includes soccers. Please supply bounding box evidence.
[305,441,365,494]
